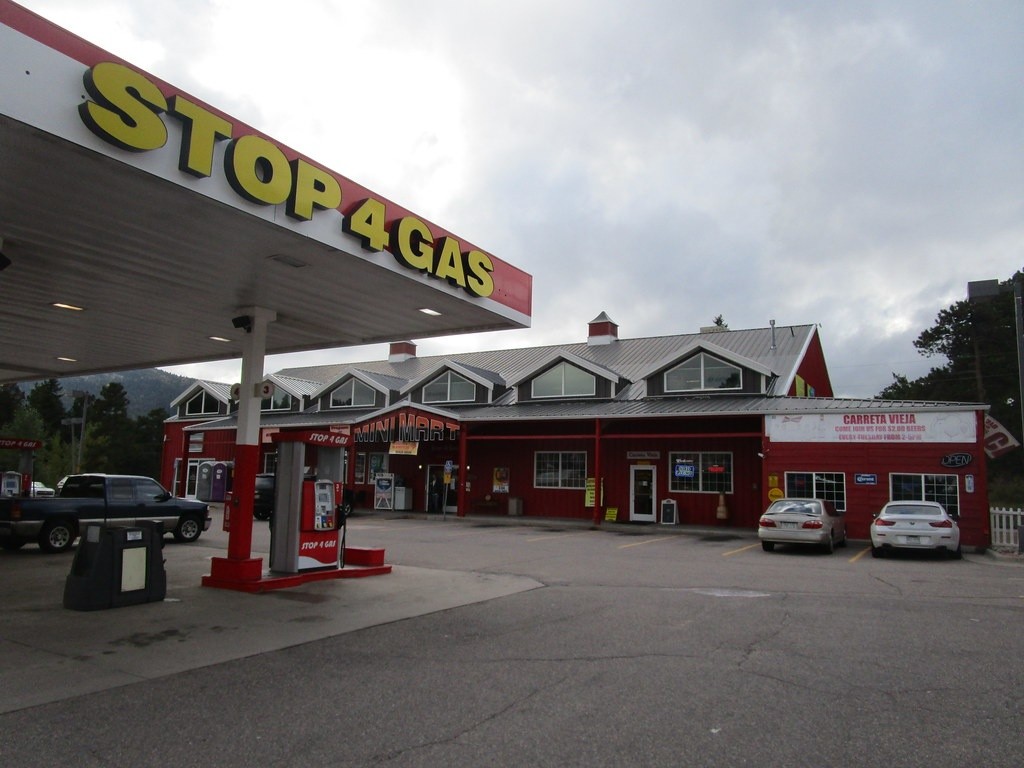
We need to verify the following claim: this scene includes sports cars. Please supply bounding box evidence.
[870,500,962,561]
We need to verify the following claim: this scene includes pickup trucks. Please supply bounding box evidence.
[0,474,213,553]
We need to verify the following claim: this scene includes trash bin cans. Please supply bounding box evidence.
[508,497,523,515]
[432,492,442,512]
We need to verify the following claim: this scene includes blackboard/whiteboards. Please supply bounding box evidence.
[661,500,681,525]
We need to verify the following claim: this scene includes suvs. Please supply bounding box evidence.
[253,473,357,521]
[57,474,105,498]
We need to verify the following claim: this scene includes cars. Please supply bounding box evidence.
[31,481,55,498]
[757,497,847,553]
